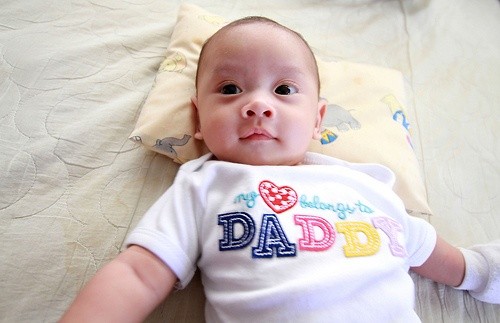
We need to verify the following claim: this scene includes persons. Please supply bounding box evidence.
[55,16,500,323]
